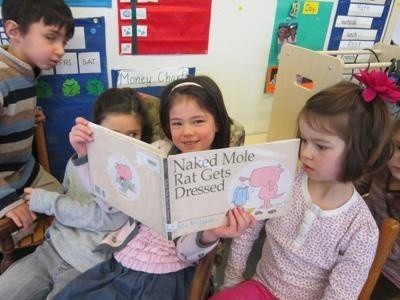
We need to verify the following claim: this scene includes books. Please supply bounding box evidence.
[86,122,300,241]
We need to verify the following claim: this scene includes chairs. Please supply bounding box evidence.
[0,106,55,275]
[356,216,400,300]
[132,93,245,300]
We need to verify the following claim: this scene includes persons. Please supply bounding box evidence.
[1,87,155,299]
[351,117,400,299]
[0,0,74,259]
[211,68,400,299]
[288,25,297,42]
[50,74,257,299]
[278,28,287,44]
